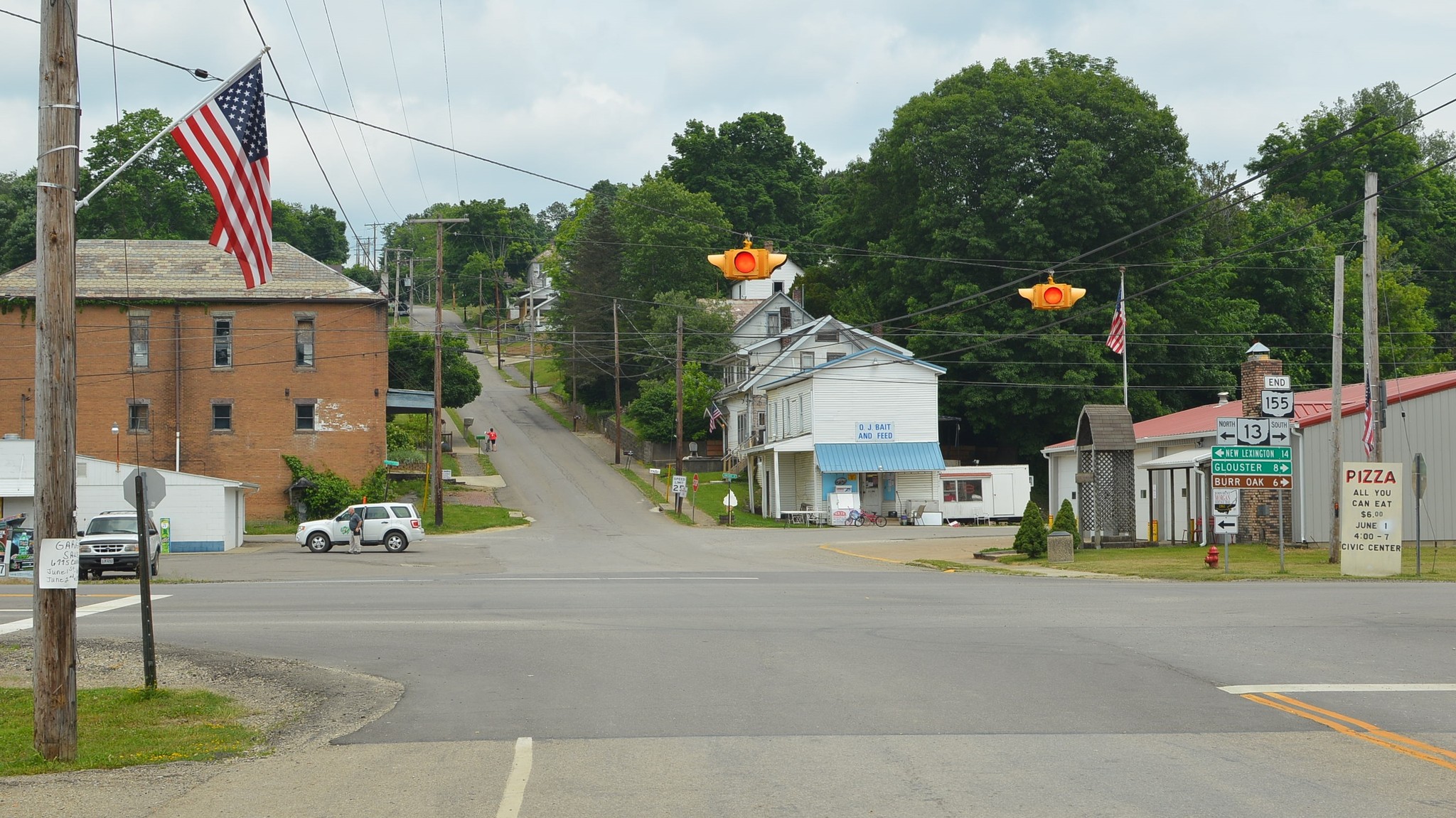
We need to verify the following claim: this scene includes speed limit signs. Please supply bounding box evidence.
[672,475,686,494]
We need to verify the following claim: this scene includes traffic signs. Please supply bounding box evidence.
[1211,460,1292,475]
[1216,417,1291,446]
[1211,474,1293,489]
[1212,445,1292,460]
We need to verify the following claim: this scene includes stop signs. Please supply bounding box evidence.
[693,474,699,492]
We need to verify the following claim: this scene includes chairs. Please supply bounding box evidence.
[689,442,699,457]
[806,505,824,528]
[790,507,805,524]
[906,505,926,526]
[1190,516,1216,544]
[972,506,991,527]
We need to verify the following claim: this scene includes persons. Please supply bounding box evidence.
[486,428,497,451]
[345,507,362,554]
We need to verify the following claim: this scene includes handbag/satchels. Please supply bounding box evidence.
[354,529,360,535]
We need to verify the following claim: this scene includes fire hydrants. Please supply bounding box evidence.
[1205,545,1219,569]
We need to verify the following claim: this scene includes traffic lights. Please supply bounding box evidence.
[1019,277,1087,310]
[708,239,788,280]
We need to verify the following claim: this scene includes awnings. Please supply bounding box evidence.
[815,443,947,472]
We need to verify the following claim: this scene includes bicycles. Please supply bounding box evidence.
[855,506,887,527]
[845,506,871,527]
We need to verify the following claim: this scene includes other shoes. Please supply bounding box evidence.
[345,551,353,554]
[354,552,360,554]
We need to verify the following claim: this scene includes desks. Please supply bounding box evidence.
[780,510,827,529]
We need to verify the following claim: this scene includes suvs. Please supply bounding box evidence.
[78,510,161,580]
[296,503,424,553]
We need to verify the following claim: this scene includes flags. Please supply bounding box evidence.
[709,403,722,433]
[1105,284,1127,355]
[1362,369,1377,457]
[173,59,274,290]
